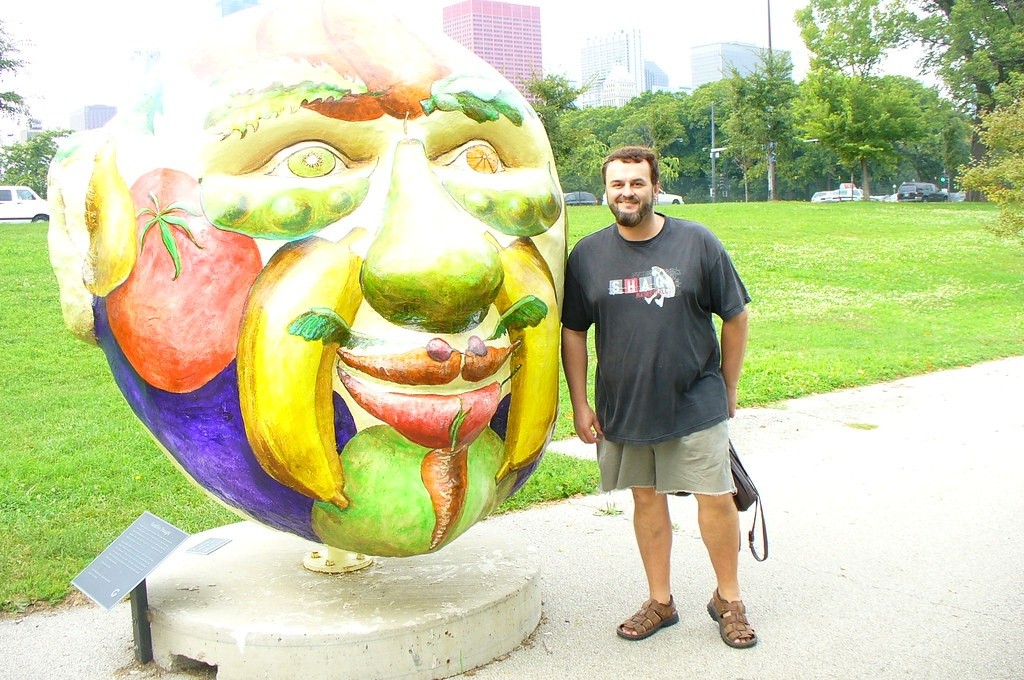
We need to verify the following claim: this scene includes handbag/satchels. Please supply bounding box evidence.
[728,438,758,511]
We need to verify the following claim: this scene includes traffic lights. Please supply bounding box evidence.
[940,175,945,183]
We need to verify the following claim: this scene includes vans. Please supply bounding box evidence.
[0,185,50,223]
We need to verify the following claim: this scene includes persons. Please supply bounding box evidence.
[560,146,758,650]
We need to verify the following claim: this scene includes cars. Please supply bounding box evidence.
[600,185,684,206]
[563,190,597,205]
[810,182,897,202]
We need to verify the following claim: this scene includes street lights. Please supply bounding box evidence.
[702,100,724,204]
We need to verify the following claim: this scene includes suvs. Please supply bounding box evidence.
[895,182,948,204]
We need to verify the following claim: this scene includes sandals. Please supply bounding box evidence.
[616,593,680,640]
[708,585,758,650]
